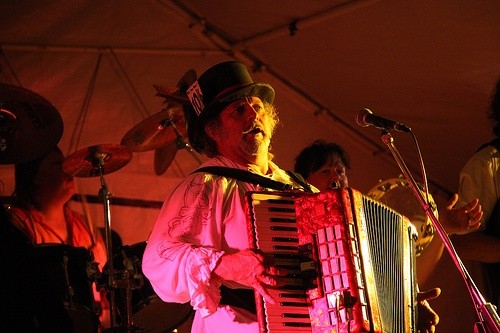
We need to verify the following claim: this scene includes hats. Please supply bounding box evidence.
[182,61,275,154]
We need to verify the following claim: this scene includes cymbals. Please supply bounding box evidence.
[151,145,178,177]
[120,104,194,153]
[64,142,131,180]
[364,175,438,259]
[1,82,65,165]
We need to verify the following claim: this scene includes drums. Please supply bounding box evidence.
[1,242,97,330]
[98,242,192,332]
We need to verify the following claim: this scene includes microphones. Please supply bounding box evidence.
[355,107,412,133]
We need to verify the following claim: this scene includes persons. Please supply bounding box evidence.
[449,78,500,318]
[9,139,111,333]
[294,139,483,289]
[141,61,442,333]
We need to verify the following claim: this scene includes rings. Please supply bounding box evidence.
[467,213,471,220]
